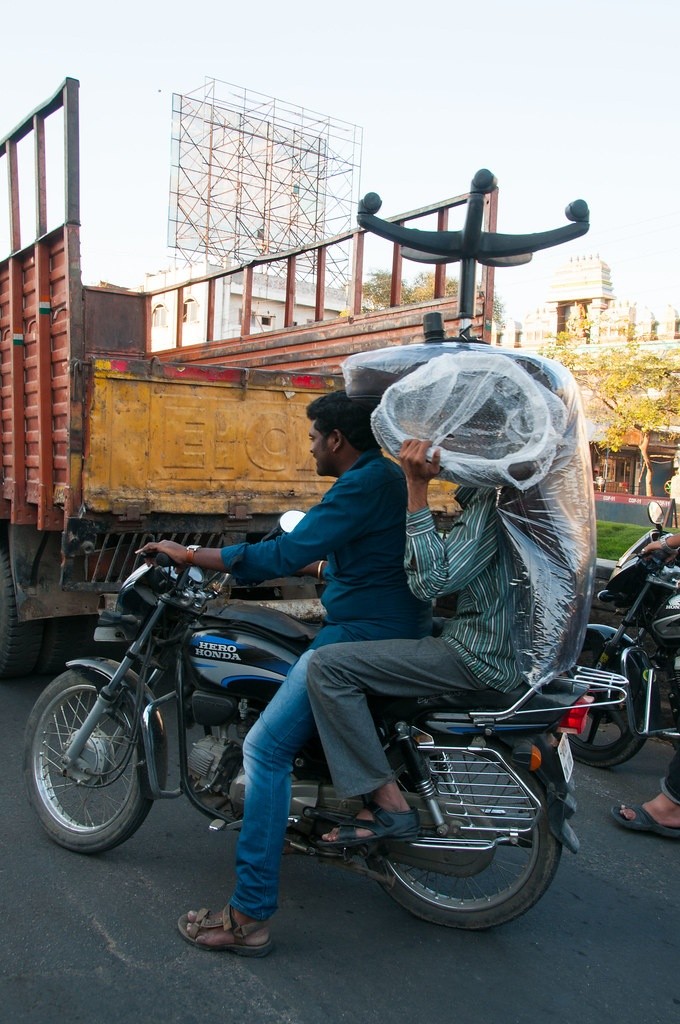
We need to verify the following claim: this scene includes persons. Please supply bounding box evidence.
[304,438,533,851]
[609,529,680,837]
[131,391,434,958]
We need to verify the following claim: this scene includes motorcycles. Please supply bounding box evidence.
[557,498,680,766]
[19,543,632,933]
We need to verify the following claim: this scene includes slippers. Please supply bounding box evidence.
[610,804,679,840]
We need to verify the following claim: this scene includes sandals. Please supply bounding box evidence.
[177,903,274,958]
[316,802,418,848]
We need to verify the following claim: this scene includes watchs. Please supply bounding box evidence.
[184,544,201,566]
[659,532,680,553]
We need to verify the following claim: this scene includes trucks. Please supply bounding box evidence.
[0,72,503,694]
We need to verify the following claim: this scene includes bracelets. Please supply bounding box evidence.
[316,559,324,580]
